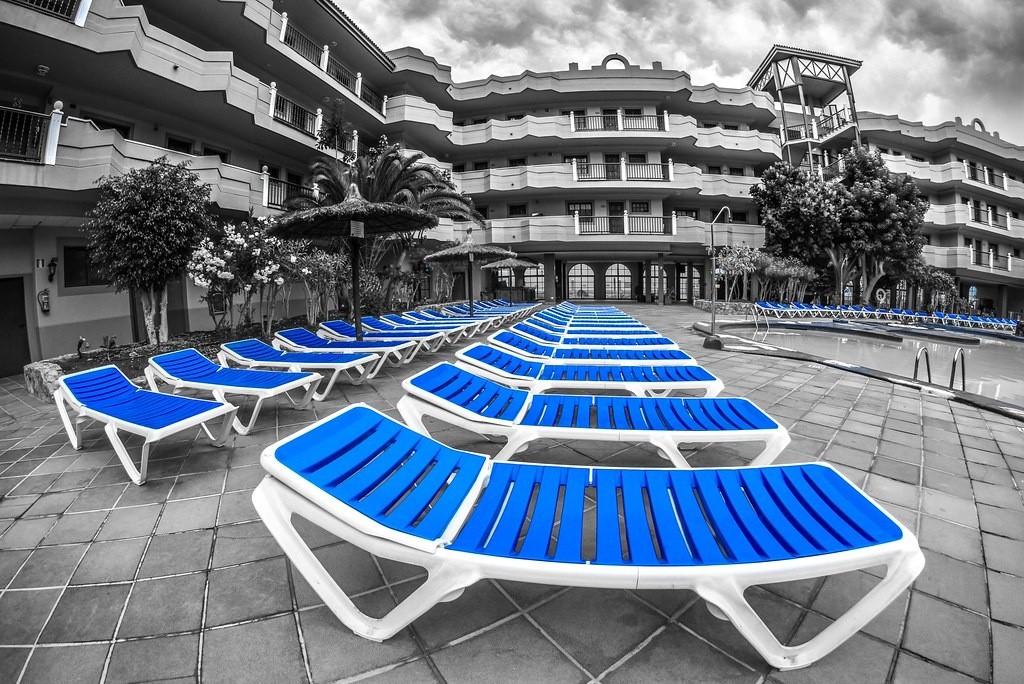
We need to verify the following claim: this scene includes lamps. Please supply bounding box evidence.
[47,259,58,282]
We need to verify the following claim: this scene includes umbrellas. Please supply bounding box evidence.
[423,228,518,317]
[479,257,540,307]
[263,183,441,341]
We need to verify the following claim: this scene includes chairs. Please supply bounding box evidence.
[753,300,1018,334]
[52,364,240,485]
[252,402,927,672]
[453,343,725,397]
[143,347,324,435]
[395,360,792,472]
[217,338,382,402]
[216,297,727,365]
